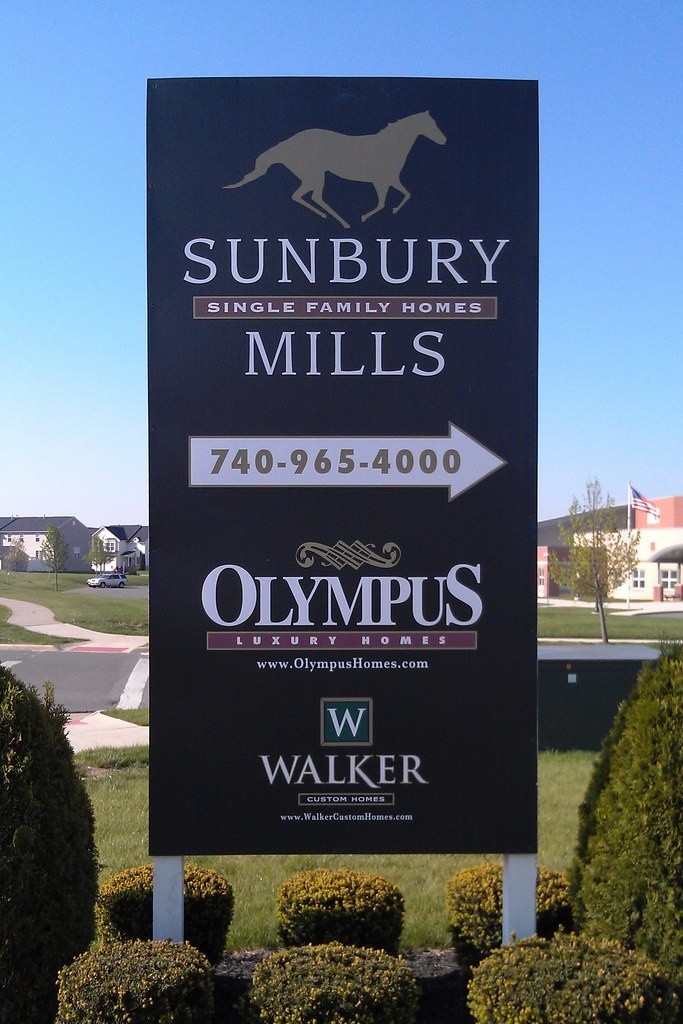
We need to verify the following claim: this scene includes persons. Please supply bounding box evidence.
[113,564,123,573]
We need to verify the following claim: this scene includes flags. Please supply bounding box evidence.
[631,486,660,517]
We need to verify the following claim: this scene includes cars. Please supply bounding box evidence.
[87,573,128,588]
